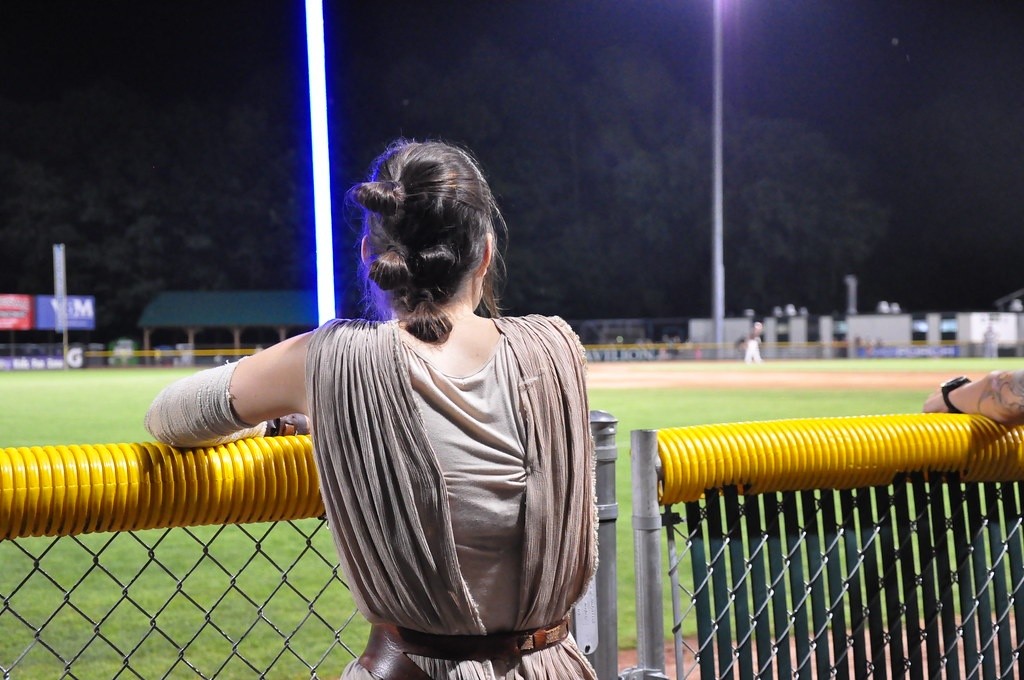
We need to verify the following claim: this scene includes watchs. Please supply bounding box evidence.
[941,375,972,413]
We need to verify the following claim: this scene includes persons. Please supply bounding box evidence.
[142,141,600,680]
[741,321,763,366]
[922,369,1024,425]
